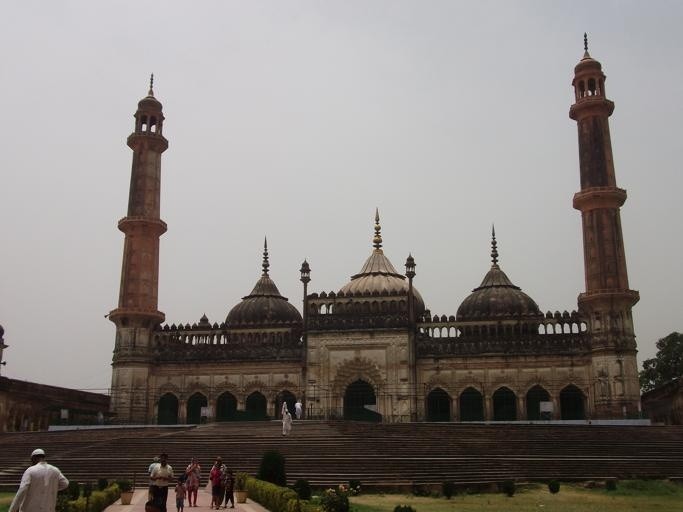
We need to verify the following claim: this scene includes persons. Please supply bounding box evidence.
[281,402,287,419]
[283,409,293,437]
[145,453,234,512]
[7,448,69,512]
[201,403,208,425]
[295,400,302,420]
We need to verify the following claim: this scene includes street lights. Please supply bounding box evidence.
[0,323,10,367]
[298,258,312,418]
[403,253,417,423]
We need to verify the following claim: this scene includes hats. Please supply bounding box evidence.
[31,449,45,457]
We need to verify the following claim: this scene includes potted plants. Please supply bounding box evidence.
[115,476,135,506]
[234,468,249,504]
